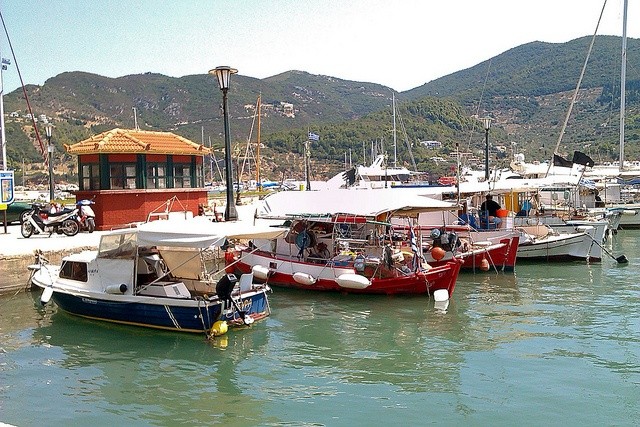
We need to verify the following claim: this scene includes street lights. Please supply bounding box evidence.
[208,66,238,221]
[480,116,495,180]
[44,123,56,201]
[302,141,312,191]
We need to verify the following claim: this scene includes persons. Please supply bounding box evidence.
[481,194,503,232]
[430,228,461,252]
[216,274,236,310]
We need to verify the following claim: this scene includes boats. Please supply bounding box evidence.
[394,186,520,270]
[502,164,640,260]
[225,191,463,298]
[31,220,290,333]
[354,166,425,189]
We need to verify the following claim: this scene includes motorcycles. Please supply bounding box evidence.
[21,202,79,238]
[63,195,96,233]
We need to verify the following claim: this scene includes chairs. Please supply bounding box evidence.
[478,209,497,230]
[231,272,253,293]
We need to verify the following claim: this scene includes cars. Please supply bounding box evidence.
[0,201,34,224]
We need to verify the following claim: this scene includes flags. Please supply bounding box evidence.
[308,133,319,141]
